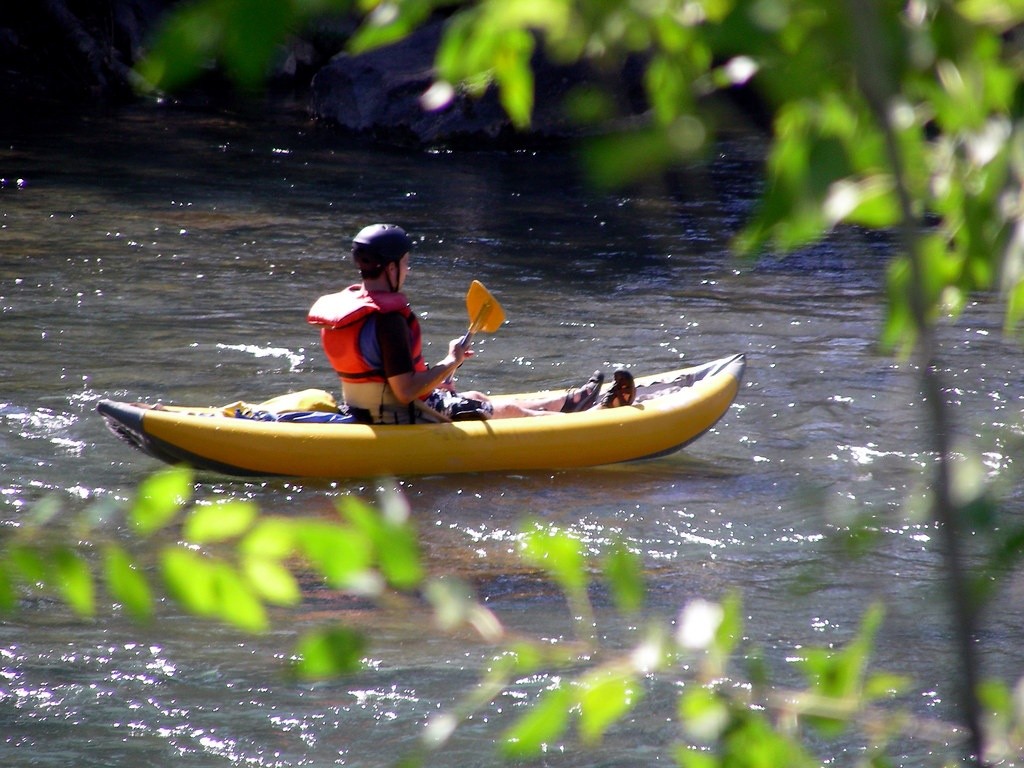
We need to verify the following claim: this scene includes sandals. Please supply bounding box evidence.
[601,368,637,410]
[559,369,604,413]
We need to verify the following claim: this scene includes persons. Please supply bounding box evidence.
[320,220,640,428]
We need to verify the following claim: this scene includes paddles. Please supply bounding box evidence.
[444,278,506,385]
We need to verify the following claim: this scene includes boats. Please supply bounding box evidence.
[98,354,749,481]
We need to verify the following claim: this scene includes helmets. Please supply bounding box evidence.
[350,223,413,269]
